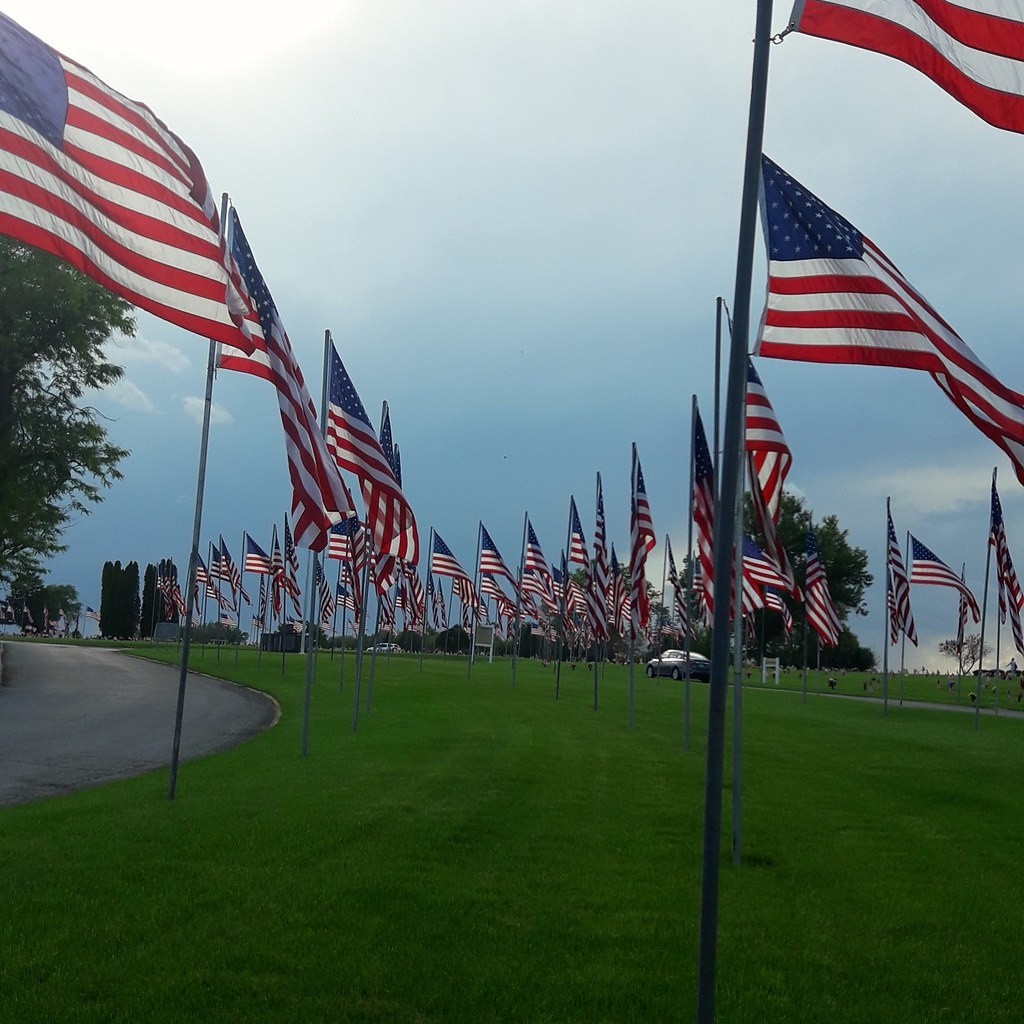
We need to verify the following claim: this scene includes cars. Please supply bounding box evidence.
[646,650,711,683]
[365,643,401,652]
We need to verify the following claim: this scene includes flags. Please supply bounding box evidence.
[2,13,256,352]
[1,327,1024,657]
[215,204,356,551]
[784,0,1024,134]
[748,148,1024,484]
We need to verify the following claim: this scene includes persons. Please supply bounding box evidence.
[1005,657,1018,679]
